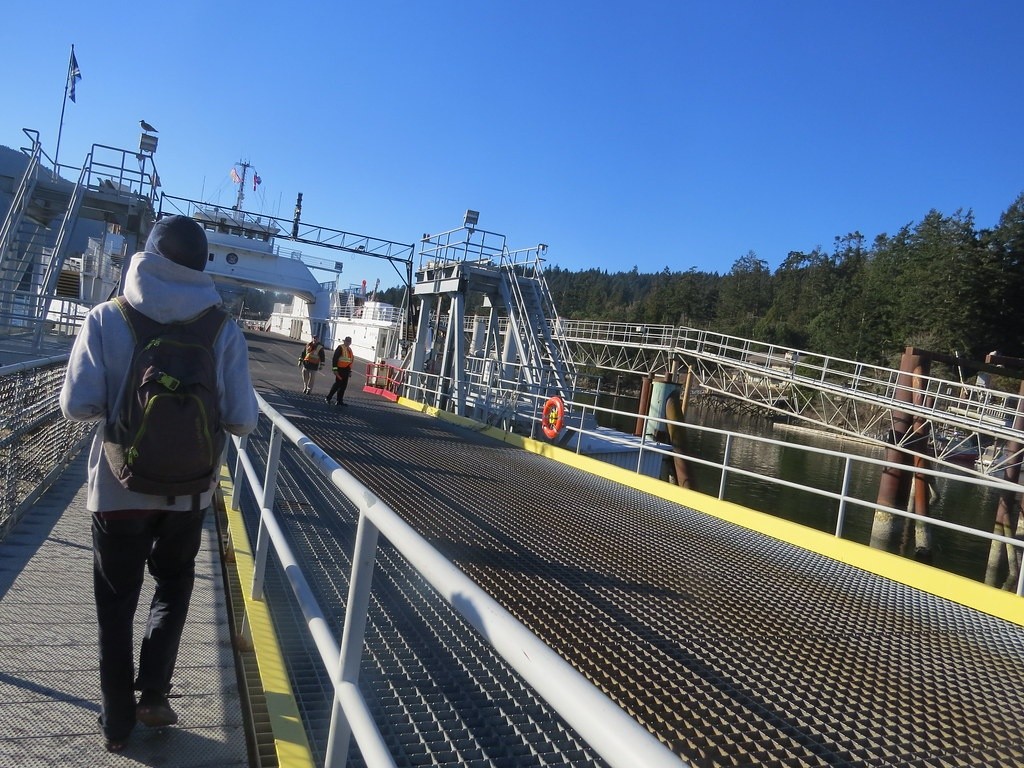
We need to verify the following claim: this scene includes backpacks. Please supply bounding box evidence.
[102,294,231,521]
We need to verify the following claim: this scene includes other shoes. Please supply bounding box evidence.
[325,397,331,405]
[303,389,306,394]
[305,389,312,396]
[97,712,133,753]
[134,701,179,726]
[335,401,348,407]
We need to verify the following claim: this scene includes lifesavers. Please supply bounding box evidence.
[542,397,564,439]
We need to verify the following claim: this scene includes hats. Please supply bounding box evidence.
[146,215,208,272]
[312,334,321,338]
[342,337,351,342]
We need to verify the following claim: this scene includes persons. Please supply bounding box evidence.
[59,214,259,751]
[297,335,325,395]
[324,337,354,405]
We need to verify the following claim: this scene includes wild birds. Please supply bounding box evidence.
[139,119,158,134]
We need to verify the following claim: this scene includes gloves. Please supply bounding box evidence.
[348,371,352,378]
[297,360,303,366]
[333,370,340,374]
[318,364,324,370]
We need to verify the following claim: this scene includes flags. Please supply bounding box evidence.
[67,51,82,104]
[231,169,243,184]
[253,175,257,191]
